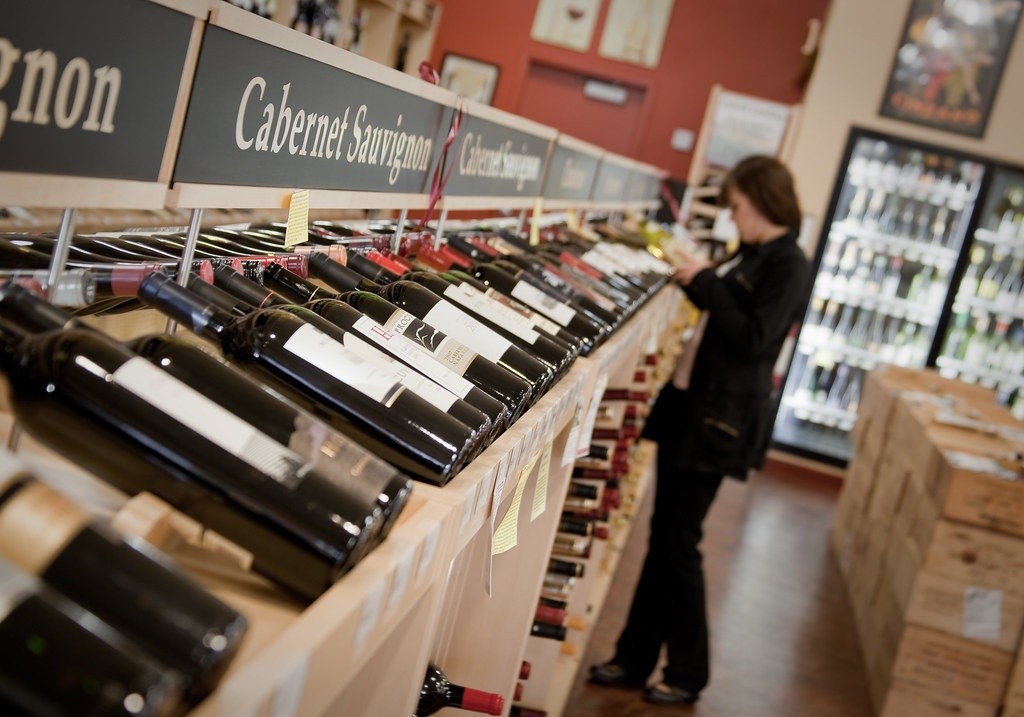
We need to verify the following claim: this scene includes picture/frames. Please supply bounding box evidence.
[879,0,1024,140]
[438,52,500,107]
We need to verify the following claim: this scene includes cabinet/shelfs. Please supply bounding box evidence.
[0,210,702,717]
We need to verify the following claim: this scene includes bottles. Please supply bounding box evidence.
[0,218,696,717]
[789,137,1024,441]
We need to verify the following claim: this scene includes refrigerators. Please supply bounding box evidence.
[769,125,1024,477]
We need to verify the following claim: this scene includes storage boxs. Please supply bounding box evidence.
[832,367,1024,717]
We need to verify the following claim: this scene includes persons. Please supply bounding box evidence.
[589,152,817,707]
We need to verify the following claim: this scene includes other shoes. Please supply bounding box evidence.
[642,681,700,706]
[588,660,646,692]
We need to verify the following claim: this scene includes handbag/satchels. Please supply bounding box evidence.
[633,377,765,483]
